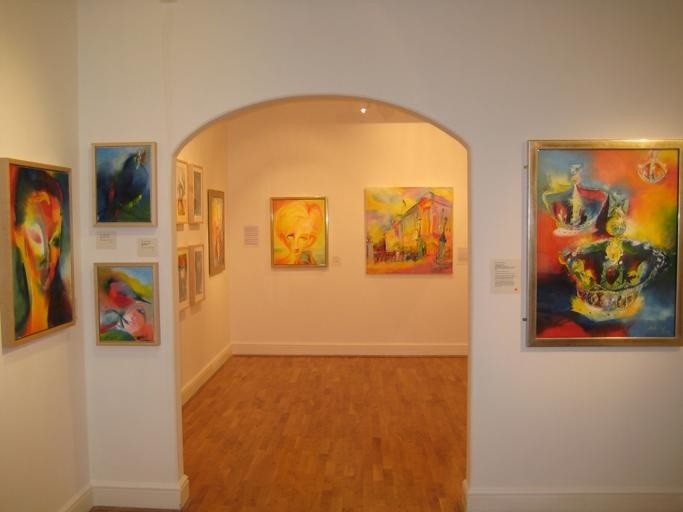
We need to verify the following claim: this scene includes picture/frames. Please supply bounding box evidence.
[0,157,77,351]
[92,262,160,346]
[90,142,157,227]
[526,137,683,348]
[172,160,227,312]
[269,196,329,270]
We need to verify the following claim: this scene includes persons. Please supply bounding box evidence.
[8,162,72,342]
[270,201,323,264]
